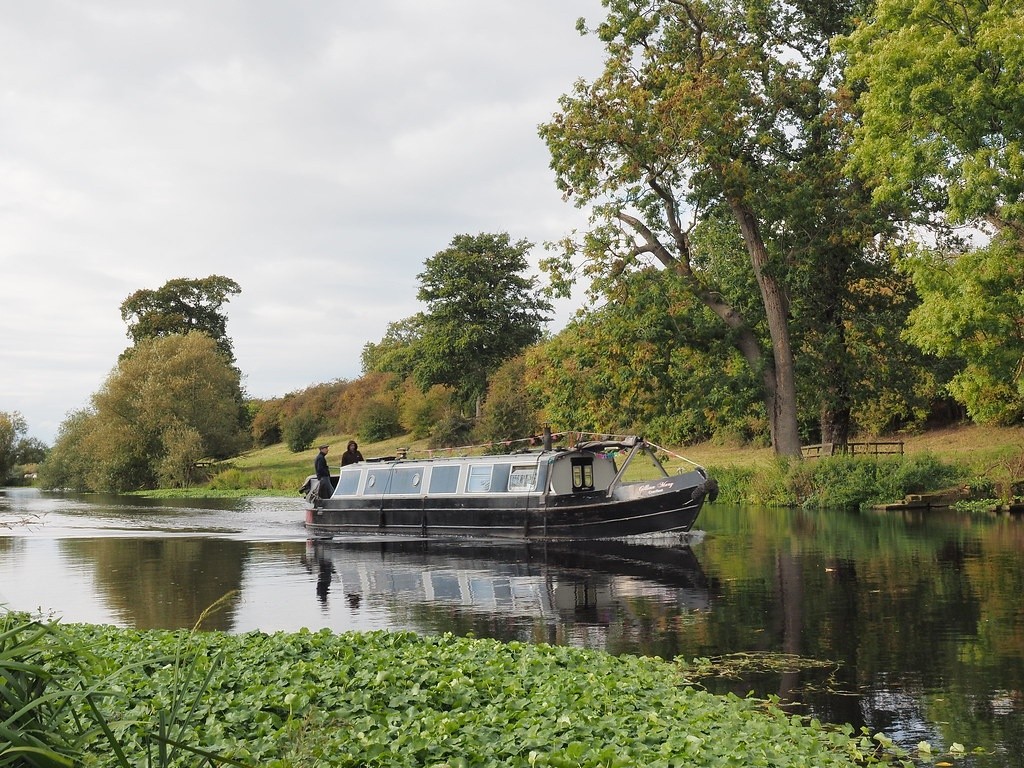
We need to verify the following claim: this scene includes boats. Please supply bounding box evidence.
[304,529,723,622]
[302,421,712,542]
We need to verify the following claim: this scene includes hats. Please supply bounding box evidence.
[318,445,329,450]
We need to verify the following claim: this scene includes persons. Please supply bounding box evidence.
[341,441,365,467]
[315,444,335,495]
